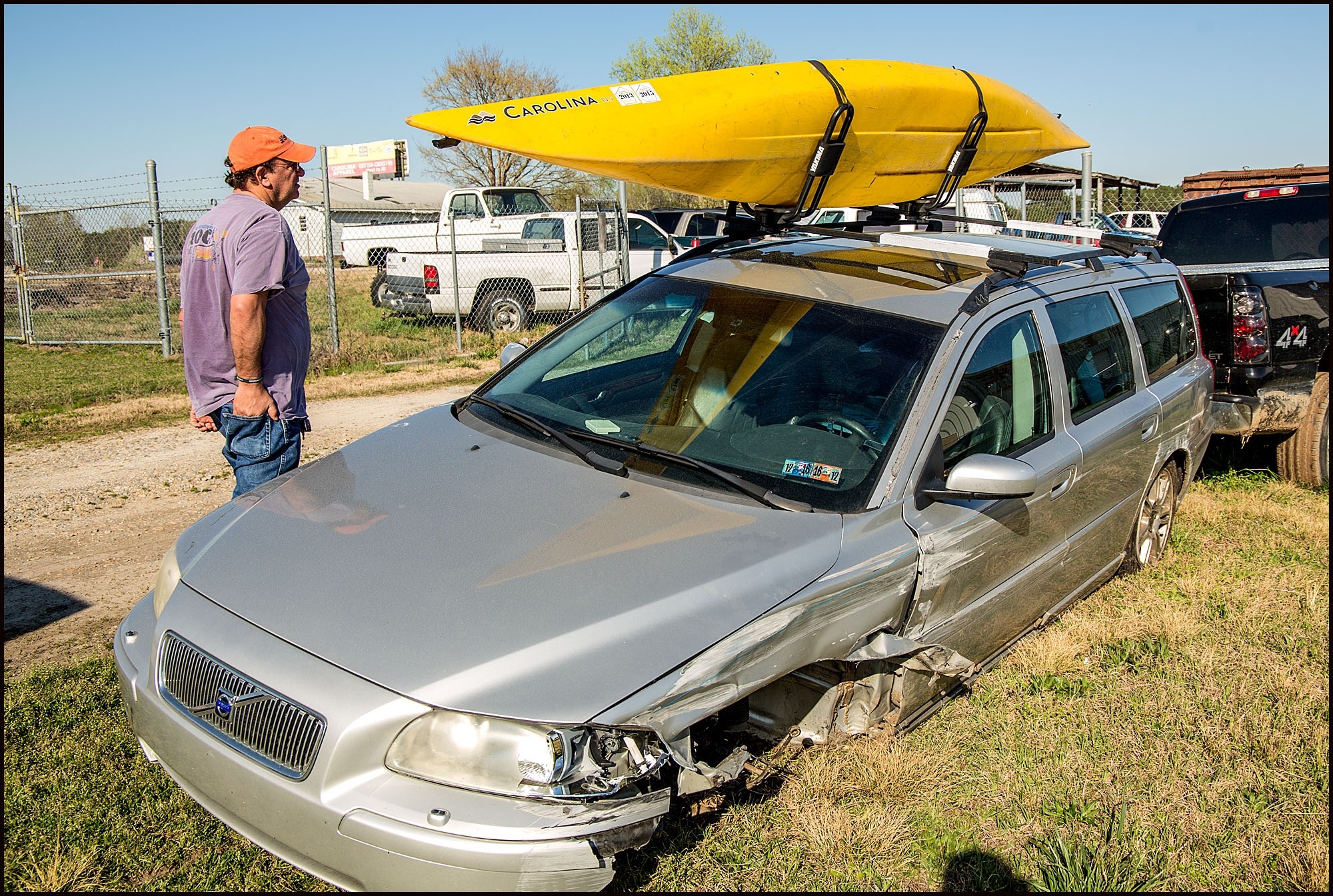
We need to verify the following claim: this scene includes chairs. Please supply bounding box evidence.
[939,378,1012,459]
[673,324,795,430]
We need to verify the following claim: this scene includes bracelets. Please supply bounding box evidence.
[236,375,263,383]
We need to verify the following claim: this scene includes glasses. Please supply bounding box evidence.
[272,163,299,171]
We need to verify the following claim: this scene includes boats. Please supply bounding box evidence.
[406,58,1092,208]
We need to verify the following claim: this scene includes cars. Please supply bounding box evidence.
[1092,210,1169,235]
[114,202,1217,896]
[626,186,1008,249]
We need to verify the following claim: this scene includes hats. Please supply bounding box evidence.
[228,126,317,173]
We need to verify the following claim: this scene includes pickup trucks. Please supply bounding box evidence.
[380,208,694,334]
[338,185,558,270]
[1000,213,1158,249]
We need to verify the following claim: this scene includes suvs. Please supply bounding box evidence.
[1144,179,1329,490]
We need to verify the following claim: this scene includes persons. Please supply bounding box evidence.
[180,126,315,501]
